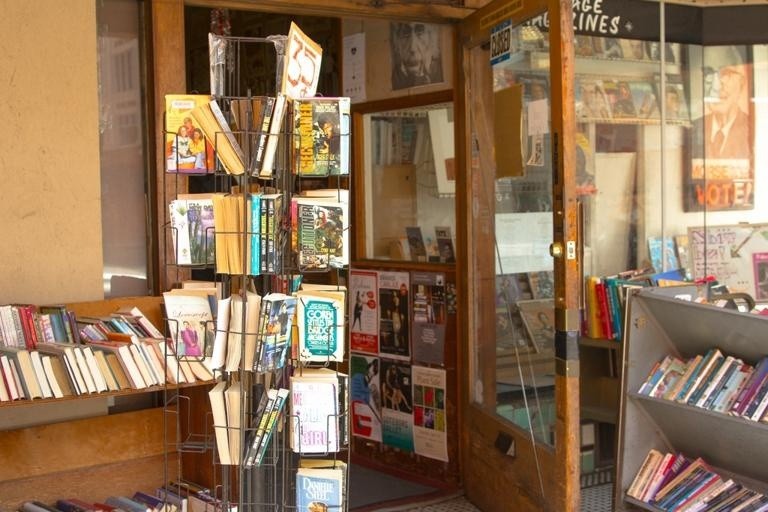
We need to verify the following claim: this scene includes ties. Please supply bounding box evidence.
[712,126,724,158]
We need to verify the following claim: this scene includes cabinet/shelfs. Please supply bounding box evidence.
[607,291,768,512]
[3,294,185,509]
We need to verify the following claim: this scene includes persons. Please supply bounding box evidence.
[392,19,442,89]
[398,282,408,347]
[382,363,411,413]
[350,359,381,423]
[691,46,750,159]
[351,291,368,331]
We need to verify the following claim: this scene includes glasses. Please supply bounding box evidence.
[704,67,743,83]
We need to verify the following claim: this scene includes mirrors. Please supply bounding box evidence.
[342,82,466,279]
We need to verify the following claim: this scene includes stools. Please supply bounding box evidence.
[571,371,622,461]
